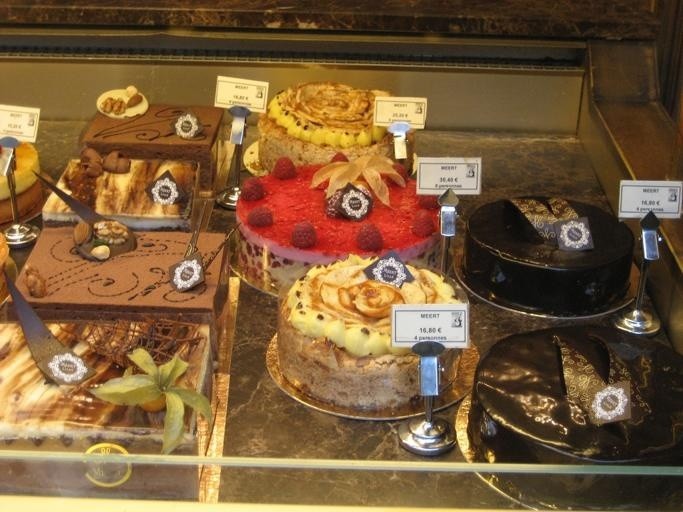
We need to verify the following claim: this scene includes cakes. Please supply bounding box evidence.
[1,81,683,511]
